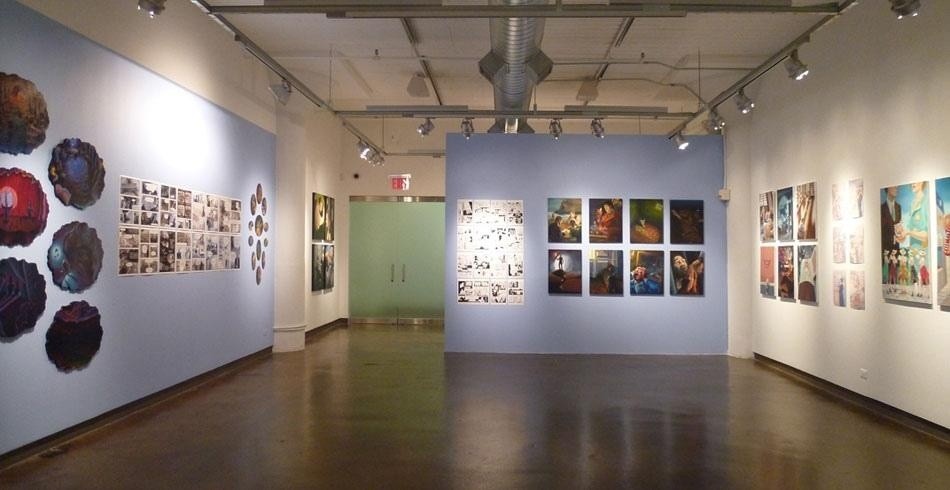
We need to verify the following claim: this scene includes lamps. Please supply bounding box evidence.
[665,0,921,150]
[334,111,694,137]
[137,0,387,169]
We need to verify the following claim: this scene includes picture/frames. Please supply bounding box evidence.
[116,174,243,276]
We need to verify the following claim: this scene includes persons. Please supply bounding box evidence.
[676,207,703,244]
[836,276,846,307]
[589,199,622,240]
[557,254,564,270]
[592,264,614,292]
[672,254,704,295]
[881,182,932,299]
[548,200,581,243]
[312,193,334,291]
[632,265,664,295]
[1,84,36,154]
[764,278,770,295]
